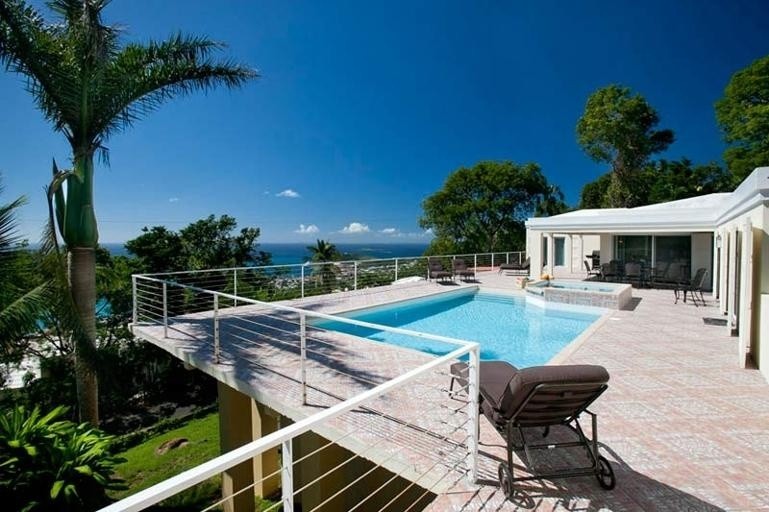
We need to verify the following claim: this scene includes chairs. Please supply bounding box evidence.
[427,257,452,285]
[497,257,530,277]
[452,259,475,282]
[449,360,617,499]
[583,250,709,307]
